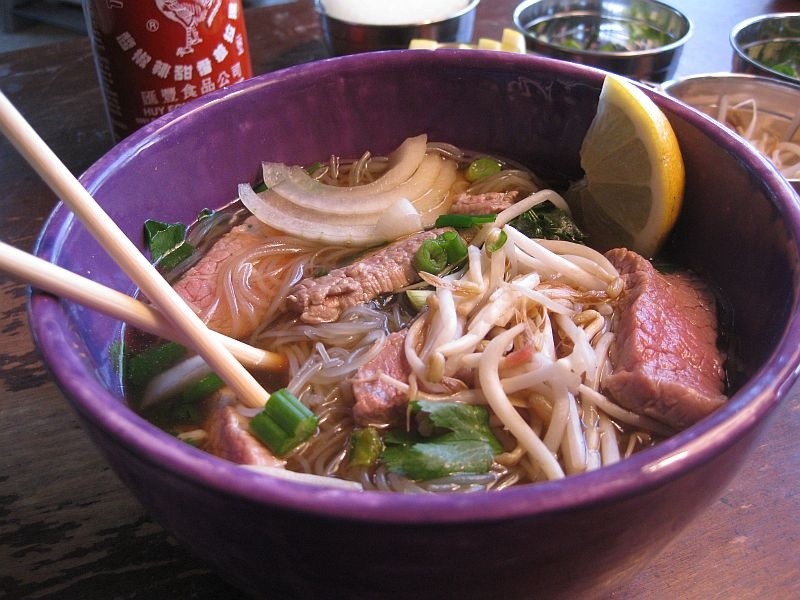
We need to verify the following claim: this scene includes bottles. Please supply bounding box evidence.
[81,0,254,146]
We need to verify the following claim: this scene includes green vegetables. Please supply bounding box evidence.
[755,58,794,80]
[510,197,731,342]
[109,206,501,481]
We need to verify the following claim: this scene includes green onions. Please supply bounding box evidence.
[559,39,616,54]
[240,153,509,453]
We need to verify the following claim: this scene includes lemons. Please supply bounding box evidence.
[406,26,528,55]
[562,79,684,261]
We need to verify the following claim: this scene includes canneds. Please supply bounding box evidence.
[83,0,253,144]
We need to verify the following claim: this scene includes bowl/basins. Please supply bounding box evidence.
[314,0,481,58]
[513,0,694,86]
[729,12,800,86]
[28,50,800,600]
[655,72,800,193]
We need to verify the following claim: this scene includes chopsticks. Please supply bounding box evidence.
[0,89,284,408]
